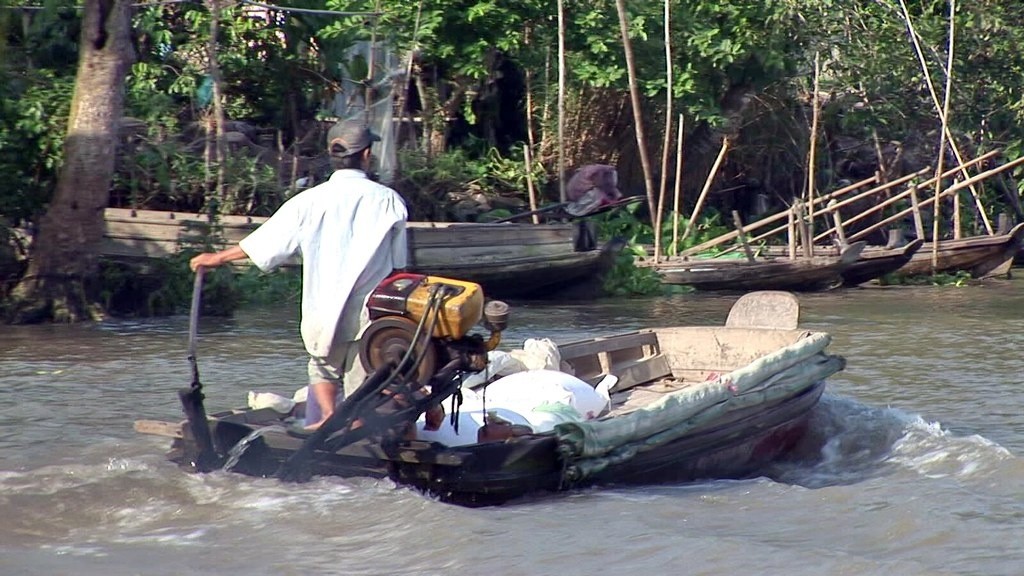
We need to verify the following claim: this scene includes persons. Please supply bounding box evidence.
[406,40,528,159]
[189,118,408,436]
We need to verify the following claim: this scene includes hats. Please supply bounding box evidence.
[327,119,381,157]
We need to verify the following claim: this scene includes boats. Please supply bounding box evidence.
[0,165,646,300]
[593,180,1024,288]
[133,264,847,510]
[656,237,866,294]
